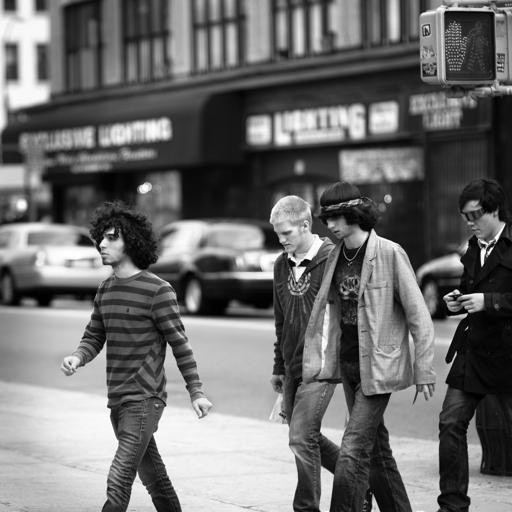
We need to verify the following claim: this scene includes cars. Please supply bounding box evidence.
[415,234,473,319]
[149,216,283,315]
[1,222,115,306]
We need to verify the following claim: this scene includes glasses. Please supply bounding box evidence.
[458,206,487,222]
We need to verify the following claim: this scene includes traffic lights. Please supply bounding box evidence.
[418,8,496,89]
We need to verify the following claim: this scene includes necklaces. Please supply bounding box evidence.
[343,230,369,266]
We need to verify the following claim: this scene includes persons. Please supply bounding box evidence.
[269,196,373,511]
[59,202,212,511]
[299,180,438,511]
[437,177,512,511]
